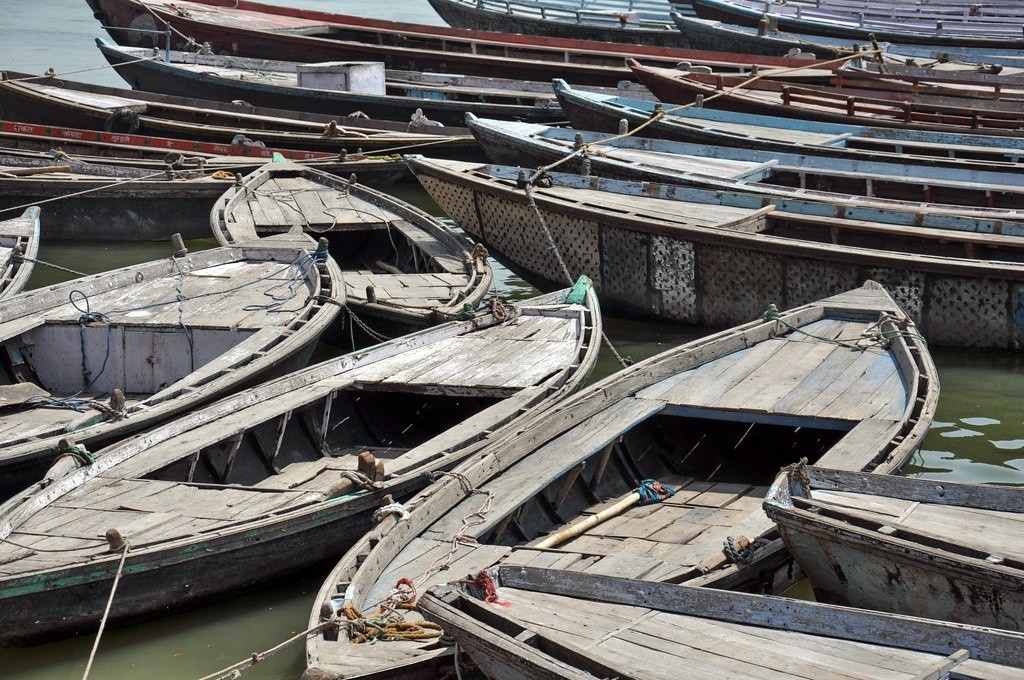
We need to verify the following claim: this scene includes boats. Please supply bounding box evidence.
[305,278,942,680]
[0,214,348,488]
[0,0,1023,364]
[0,273,603,640]
[761,456,1024,636]
[415,564,1024,680]
